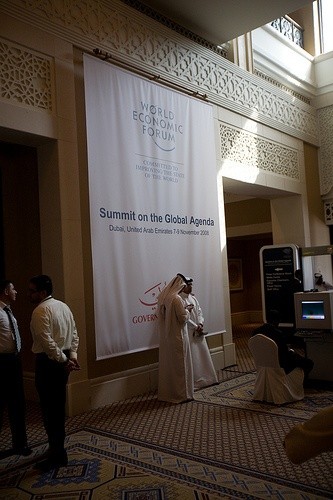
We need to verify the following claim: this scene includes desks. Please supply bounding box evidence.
[292,341,333,380]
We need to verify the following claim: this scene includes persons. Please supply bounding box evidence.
[252,309,315,381]
[0,279,33,456]
[282,269,304,293]
[178,276,220,390]
[314,273,330,291]
[29,274,81,465]
[156,273,195,403]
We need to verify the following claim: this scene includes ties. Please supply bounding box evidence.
[3,306,22,353]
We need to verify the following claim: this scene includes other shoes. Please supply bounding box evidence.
[13,446,33,456]
[46,449,68,467]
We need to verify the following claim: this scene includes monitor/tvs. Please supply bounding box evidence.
[294,291,333,329]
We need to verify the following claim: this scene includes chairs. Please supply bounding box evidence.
[247,335,304,404]
